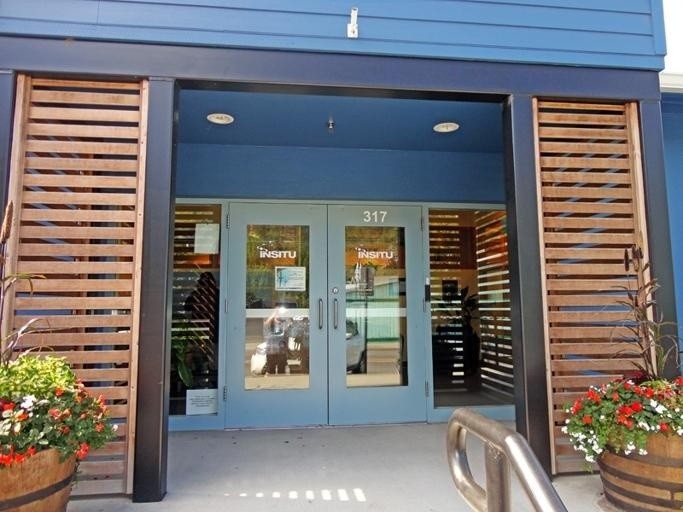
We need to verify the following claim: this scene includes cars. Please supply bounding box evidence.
[250,317,366,376]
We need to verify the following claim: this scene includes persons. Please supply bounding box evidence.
[182,272,219,389]
[262,306,293,374]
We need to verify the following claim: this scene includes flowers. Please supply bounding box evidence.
[562,244,683,475]
[0,201,118,468]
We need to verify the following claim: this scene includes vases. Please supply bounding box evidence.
[0,450,76,512]
[596,434,683,512]
